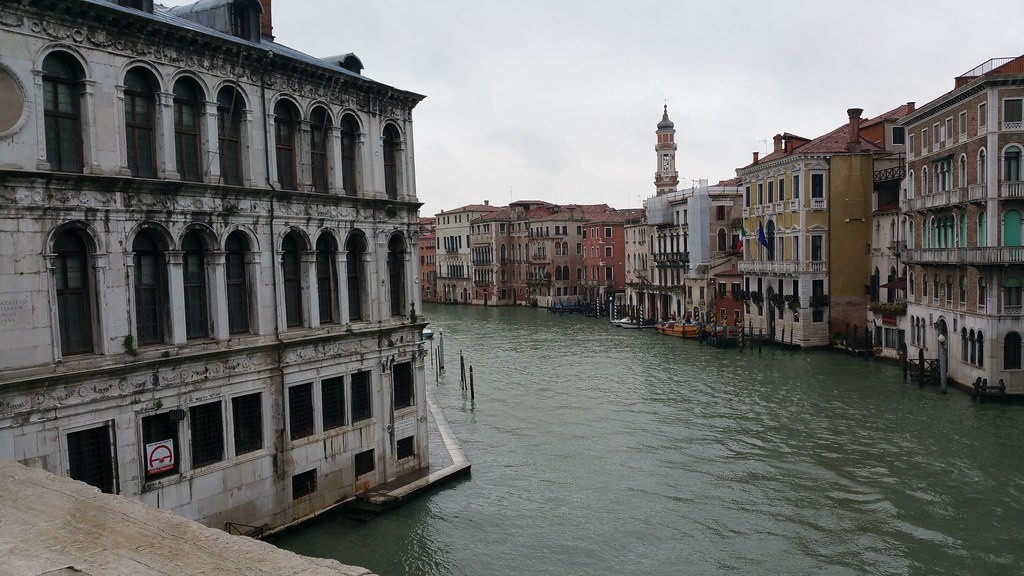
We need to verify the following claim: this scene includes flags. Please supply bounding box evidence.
[737,224,746,252]
[758,220,768,251]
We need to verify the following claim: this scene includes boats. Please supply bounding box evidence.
[701,321,739,348]
[620,322,657,328]
[656,312,703,338]
[611,315,637,326]
